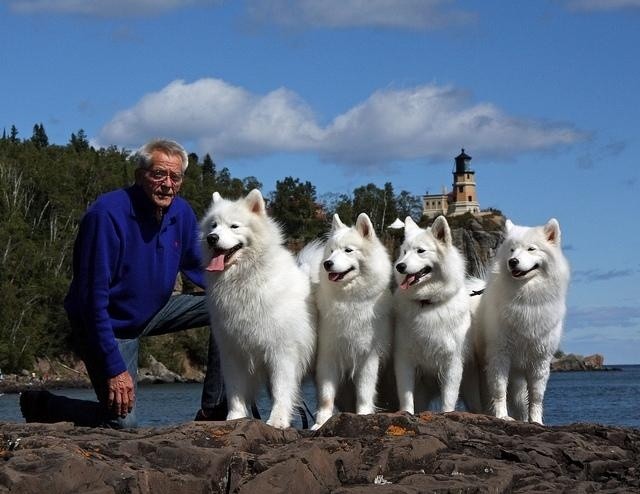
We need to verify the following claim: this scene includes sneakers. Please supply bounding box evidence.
[19,390,42,423]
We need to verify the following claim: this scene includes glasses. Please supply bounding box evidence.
[144,167,184,182]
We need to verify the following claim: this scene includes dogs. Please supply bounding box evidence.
[476,218,570,426]
[297,211,394,431]
[390,214,488,417]
[193,189,318,432]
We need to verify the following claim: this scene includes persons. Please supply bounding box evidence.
[60,135,231,429]
